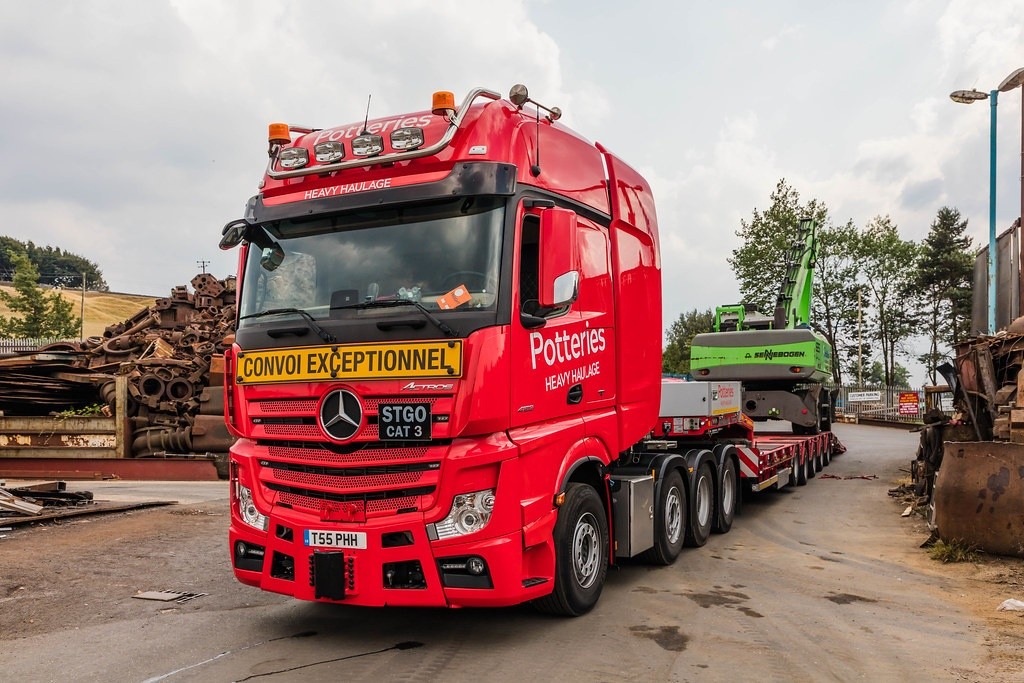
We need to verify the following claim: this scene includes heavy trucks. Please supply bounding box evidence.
[217,82,847,618]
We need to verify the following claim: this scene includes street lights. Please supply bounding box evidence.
[949,68,1024,337]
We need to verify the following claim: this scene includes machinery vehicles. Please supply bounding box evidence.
[689,217,842,437]
